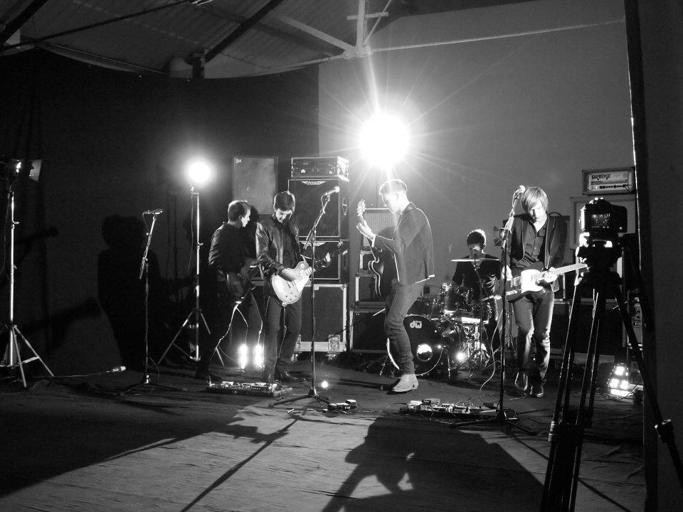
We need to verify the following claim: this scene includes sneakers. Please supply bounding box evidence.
[393,374,419,392]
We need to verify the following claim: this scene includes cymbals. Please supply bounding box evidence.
[449,256,502,264]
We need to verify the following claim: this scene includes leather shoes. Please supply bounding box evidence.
[281,372,308,385]
[515,371,530,391]
[534,384,544,397]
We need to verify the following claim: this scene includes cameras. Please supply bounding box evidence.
[575,198,627,271]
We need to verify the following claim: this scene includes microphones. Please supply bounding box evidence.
[323,186,340,196]
[144,208,169,218]
[112,366,127,372]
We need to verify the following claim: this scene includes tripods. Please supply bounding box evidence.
[155,302,226,381]
[539,271,683,511]
[1,322,56,387]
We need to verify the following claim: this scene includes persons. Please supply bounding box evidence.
[255,191,332,387]
[209,200,263,371]
[502,185,566,397]
[446,229,503,371]
[354,179,436,394]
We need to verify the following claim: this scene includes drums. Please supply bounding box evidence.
[386,317,459,376]
[439,280,460,316]
[406,296,436,322]
[456,286,475,306]
[460,300,490,325]
[416,273,442,288]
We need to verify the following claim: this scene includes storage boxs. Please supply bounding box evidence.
[295,282,350,365]
[286,230,350,283]
[345,304,395,356]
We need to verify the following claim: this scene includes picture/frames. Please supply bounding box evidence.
[570,196,640,252]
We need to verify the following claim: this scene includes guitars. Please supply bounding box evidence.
[354,199,398,310]
[269,239,350,306]
[503,261,589,302]
[224,257,267,300]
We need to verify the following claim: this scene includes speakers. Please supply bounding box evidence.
[291,239,350,285]
[289,283,351,353]
